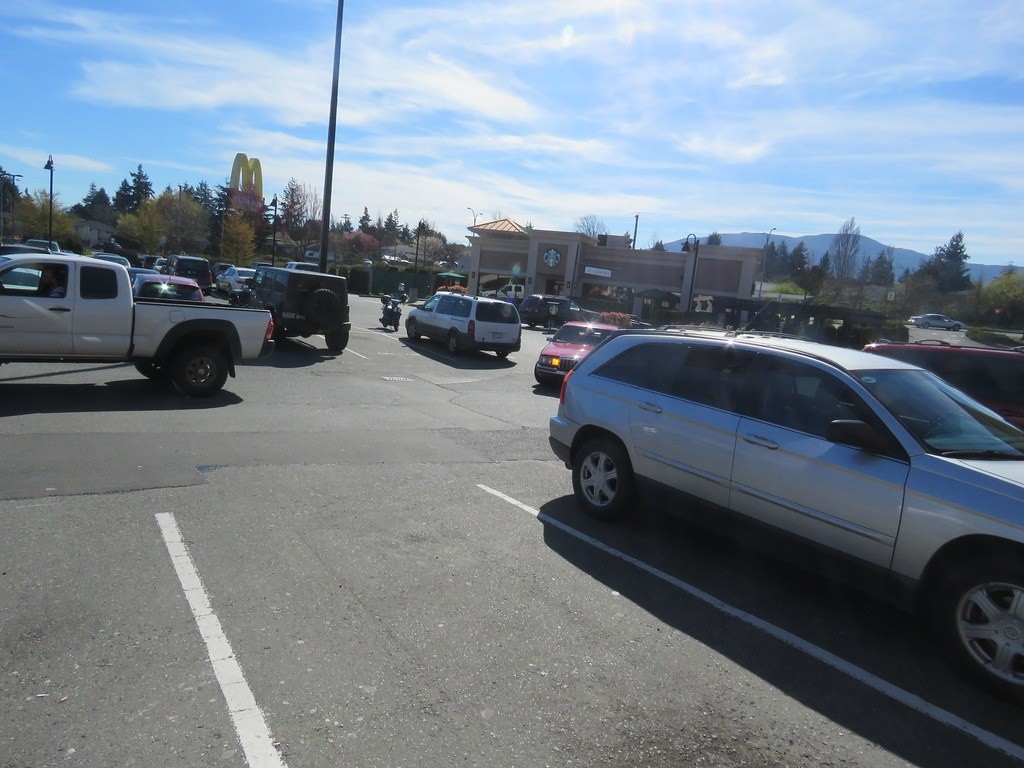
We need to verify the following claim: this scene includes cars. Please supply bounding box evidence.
[908,314,967,332]
[626,314,652,329]
[534,322,621,387]
[0,238,320,298]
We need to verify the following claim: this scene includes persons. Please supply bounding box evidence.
[39,270,65,298]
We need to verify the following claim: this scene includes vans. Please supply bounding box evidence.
[405,293,522,359]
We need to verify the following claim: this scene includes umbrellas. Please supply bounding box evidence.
[437,271,466,287]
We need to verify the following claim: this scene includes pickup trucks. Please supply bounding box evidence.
[0,254,276,399]
[482,285,525,300]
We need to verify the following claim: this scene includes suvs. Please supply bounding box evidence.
[518,295,603,330]
[859,338,1024,432]
[229,266,351,353]
[549,323,1024,709]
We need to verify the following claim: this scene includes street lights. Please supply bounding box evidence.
[6,172,23,238]
[468,207,483,237]
[682,233,700,321]
[270,194,278,265]
[758,227,776,299]
[44,154,56,251]
[413,218,425,288]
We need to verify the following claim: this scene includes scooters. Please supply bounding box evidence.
[379,295,403,332]
